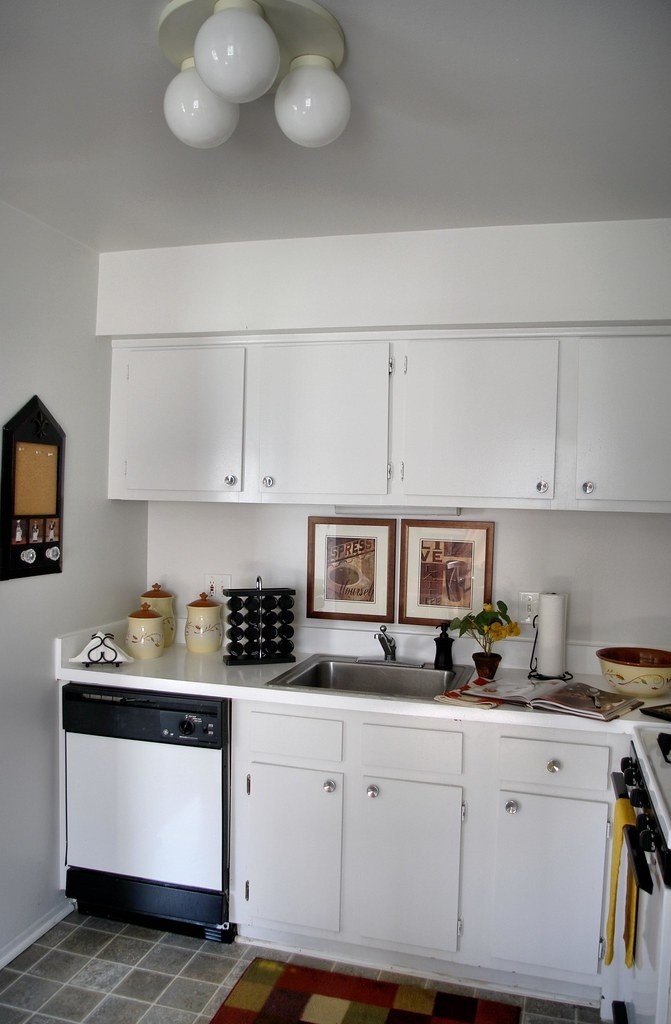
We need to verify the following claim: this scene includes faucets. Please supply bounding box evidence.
[374,625,397,661]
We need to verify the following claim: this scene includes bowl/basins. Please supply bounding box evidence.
[596,647,671,697]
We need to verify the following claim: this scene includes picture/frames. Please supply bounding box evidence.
[305,516,398,624]
[398,518,496,627]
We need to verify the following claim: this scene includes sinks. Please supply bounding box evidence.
[265,653,475,703]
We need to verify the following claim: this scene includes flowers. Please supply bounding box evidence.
[448,600,522,653]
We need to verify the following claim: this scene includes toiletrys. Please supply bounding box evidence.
[434,623,455,671]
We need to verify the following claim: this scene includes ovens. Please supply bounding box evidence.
[610,741,671,1024]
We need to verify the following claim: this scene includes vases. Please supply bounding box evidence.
[472,652,503,680]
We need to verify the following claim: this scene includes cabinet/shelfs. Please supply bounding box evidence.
[396,327,670,515]
[229,697,468,983]
[468,721,632,1009]
[106,330,397,506]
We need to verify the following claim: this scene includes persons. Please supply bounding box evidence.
[16,522,22,543]
[32,521,39,542]
[48,520,55,541]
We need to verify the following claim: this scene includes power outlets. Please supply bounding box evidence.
[205,573,233,604]
[517,591,540,626]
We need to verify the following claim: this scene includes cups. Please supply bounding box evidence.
[126,602,165,659]
[140,583,175,647]
[185,592,223,652]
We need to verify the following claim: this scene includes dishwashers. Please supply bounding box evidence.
[63,682,236,943]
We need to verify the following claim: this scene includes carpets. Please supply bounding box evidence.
[208,956,523,1024]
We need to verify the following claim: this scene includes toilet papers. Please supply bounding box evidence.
[536,592,567,678]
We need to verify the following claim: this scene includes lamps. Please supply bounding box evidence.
[156,0,352,149]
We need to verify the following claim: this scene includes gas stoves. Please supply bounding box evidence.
[657,732,671,763]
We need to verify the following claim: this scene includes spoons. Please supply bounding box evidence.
[587,688,601,707]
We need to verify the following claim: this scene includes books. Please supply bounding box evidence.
[463,677,644,721]
